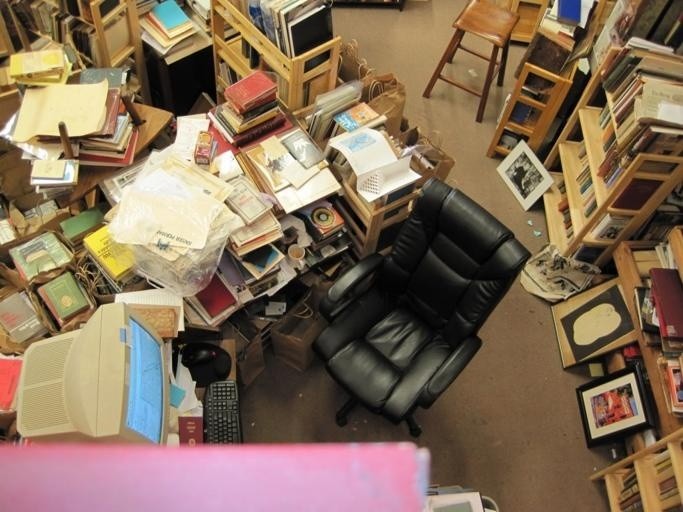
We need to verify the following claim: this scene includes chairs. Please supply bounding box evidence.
[312,175,532,437]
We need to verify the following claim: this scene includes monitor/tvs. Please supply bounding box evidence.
[16,301,170,447]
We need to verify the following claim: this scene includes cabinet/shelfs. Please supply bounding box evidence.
[488,1,683,507]
[0,1,456,511]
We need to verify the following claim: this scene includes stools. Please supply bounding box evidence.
[423,0,519,123]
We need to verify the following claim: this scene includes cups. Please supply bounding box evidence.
[286,242,306,271]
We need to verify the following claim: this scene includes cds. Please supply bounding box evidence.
[311,206,335,227]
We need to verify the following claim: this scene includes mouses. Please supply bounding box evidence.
[190,349,218,362]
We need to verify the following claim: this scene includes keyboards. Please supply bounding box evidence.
[203,380,243,445]
